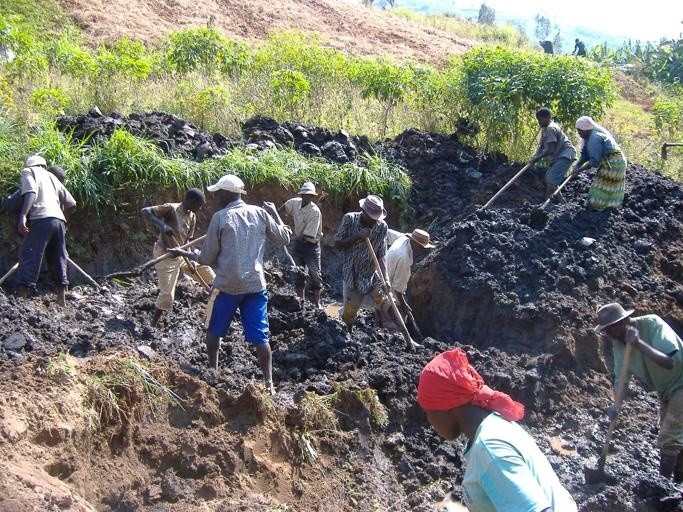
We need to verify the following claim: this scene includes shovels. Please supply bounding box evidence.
[282,244,309,279]
[584,325,635,485]
[107,232,208,278]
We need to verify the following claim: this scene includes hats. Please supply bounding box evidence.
[406,228,436,249]
[595,303,634,331]
[297,182,318,197]
[26,156,47,168]
[208,175,247,195]
[360,195,387,222]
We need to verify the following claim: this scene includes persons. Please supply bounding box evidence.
[571,116,626,211]
[335,194,426,350]
[277,182,322,303]
[572,39,586,56]
[386,229,436,325]
[594,301,683,483]
[17,154,77,308]
[529,107,577,201]
[3,166,70,292]
[417,346,577,512]
[167,174,292,395]
[140,189,221,329]
[539,40,553,54]
